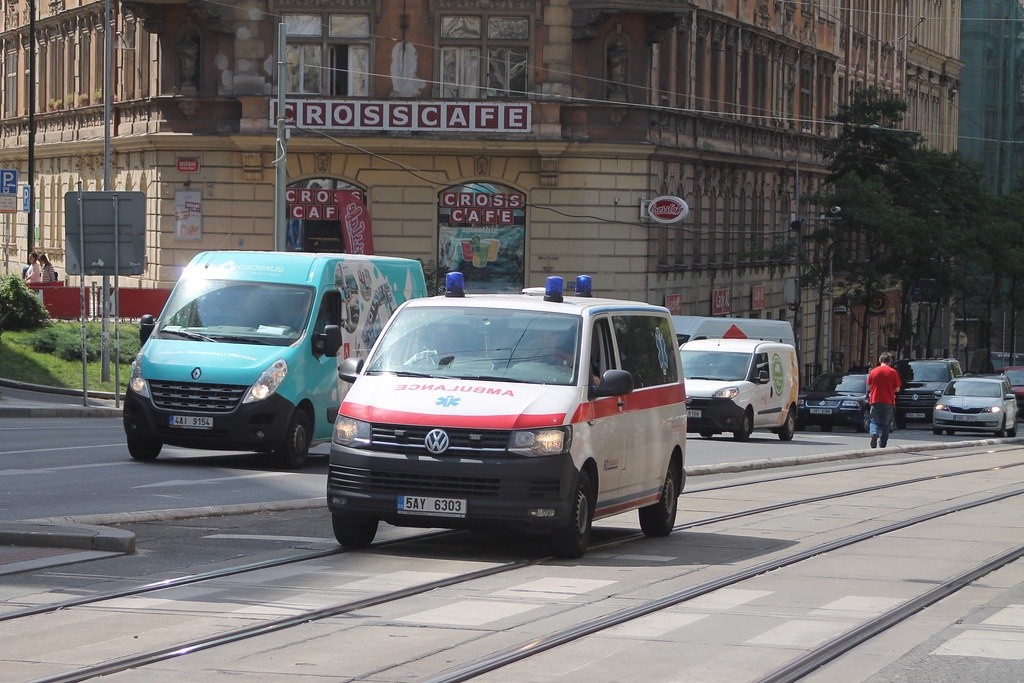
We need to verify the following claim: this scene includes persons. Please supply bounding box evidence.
[867,352,901,448]
[401,327,459,366]
[38,256,56,282]
[24,253,40,294]
[523,331,601,386]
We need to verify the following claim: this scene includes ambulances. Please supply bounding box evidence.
[325,270,688,560]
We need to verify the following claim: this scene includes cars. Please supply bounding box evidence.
[794,372,896,434]
[965,365,1024,419]
[932,372,1018,439]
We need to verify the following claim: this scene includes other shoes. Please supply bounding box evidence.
[870,432,879,448]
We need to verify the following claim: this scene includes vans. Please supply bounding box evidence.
[671,314,796,349]
[680,337,800,444]
[124,249,431,471]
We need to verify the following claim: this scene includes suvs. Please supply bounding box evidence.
[892,357,963,429]
